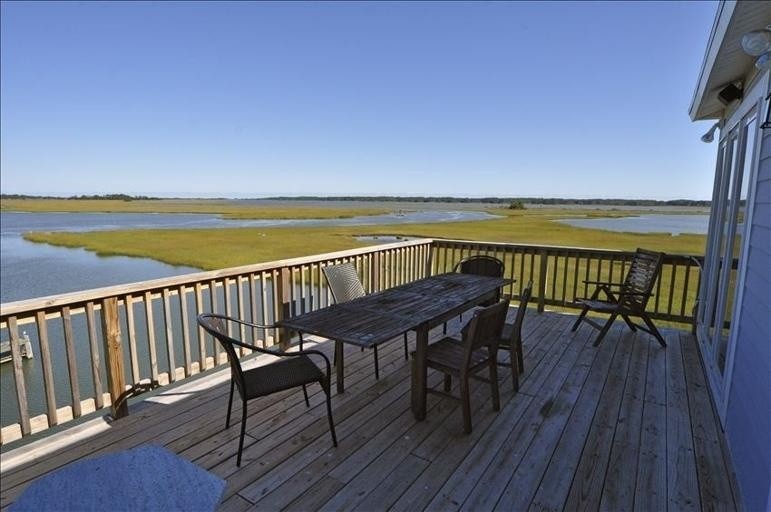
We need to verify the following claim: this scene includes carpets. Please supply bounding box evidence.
[4,441,227,511]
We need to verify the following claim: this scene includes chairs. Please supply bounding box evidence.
[196,313,338,468]
[442,255,505,335]
[321,262,408,380]
[461,280,533,393]
[570,248,667,348]
[411,298,511,434]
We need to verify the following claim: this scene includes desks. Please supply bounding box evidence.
[273,273,517,421]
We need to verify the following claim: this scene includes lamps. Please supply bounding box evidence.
[701,120,726,143]
[759,93,771,130]
[717,85,741,105]
[739,29,771,70]
